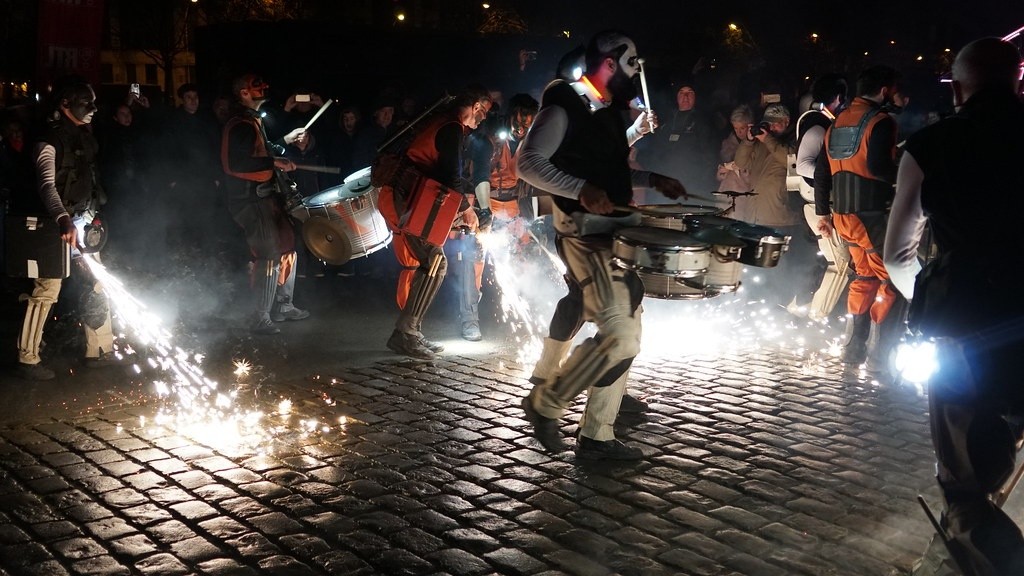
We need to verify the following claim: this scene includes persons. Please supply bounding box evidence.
[786,60,900,372]
[883,36,1024,576]
[461,94,540,341]
[377,83,494,358]
[0,49,955,277]
[222,68,312,334]
[516,32,686,459]
[16,72,115,380]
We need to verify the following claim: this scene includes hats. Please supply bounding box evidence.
[759,104,790,125]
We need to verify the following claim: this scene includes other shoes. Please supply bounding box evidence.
[19,360,56,380]
[522,393,560,452]
[393,329,444,356]
[619,393,648,412]
[251,315,280,333]
[87,349,138,367]
[387,325,434,360]
[273,305,311,322]
[575,431,642,459]
[461,317,481,341]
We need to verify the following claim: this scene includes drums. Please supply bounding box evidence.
[683,211,788,269]
[637,270,720,300]
[606,223,712,278]
[639,201,724,231]
[674,243,743,292]
[303,166,393,260]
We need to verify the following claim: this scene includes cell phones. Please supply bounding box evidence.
[724,163,733,170]
[295,95,310,102]
[131,84,140,100]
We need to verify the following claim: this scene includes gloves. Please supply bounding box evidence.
[476,209,493,231]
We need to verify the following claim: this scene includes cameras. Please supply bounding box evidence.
[749,122,770,136]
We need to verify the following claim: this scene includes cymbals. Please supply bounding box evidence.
[303,215,352,266]
[348,179,370,191]
